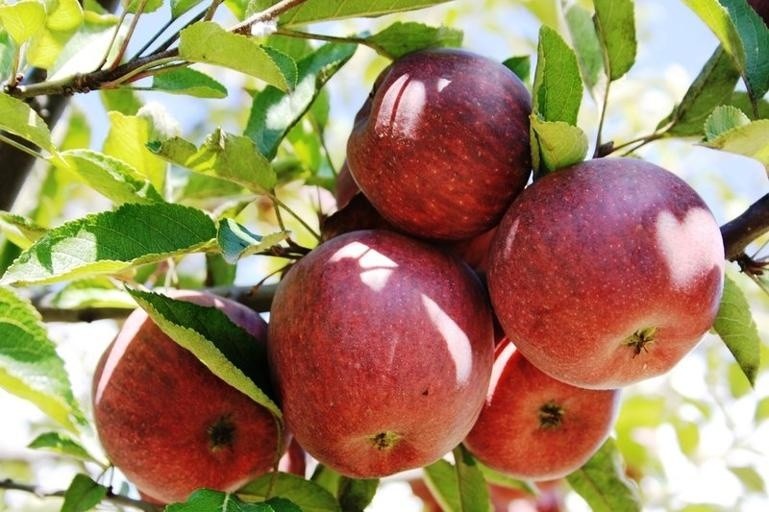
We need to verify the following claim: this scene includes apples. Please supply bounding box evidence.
[460,317,622,481]
[486,155,725,390]
[336,159,499,272]
[347,48,538,240]
[91,291,293,506]
[268,229,495,481]
